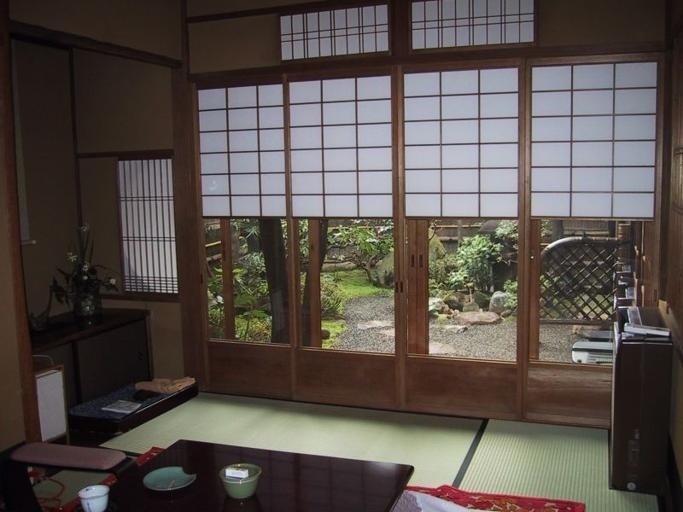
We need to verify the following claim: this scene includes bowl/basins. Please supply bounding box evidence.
[216,461,262,500]
[77,484,111,512]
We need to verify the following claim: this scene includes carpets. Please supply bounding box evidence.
[45,443,590,511]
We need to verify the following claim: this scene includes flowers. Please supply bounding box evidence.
[50,220,123,305]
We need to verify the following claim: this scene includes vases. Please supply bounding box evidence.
[72,291,104,318]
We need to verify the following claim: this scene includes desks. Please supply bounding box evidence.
[70,436,417,512]
[29,308,153,409]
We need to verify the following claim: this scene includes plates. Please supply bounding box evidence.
[143,464,199,492]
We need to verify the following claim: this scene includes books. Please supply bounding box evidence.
[100,398,142,416]
[623,322,671,336]
[620,331,672,345]
